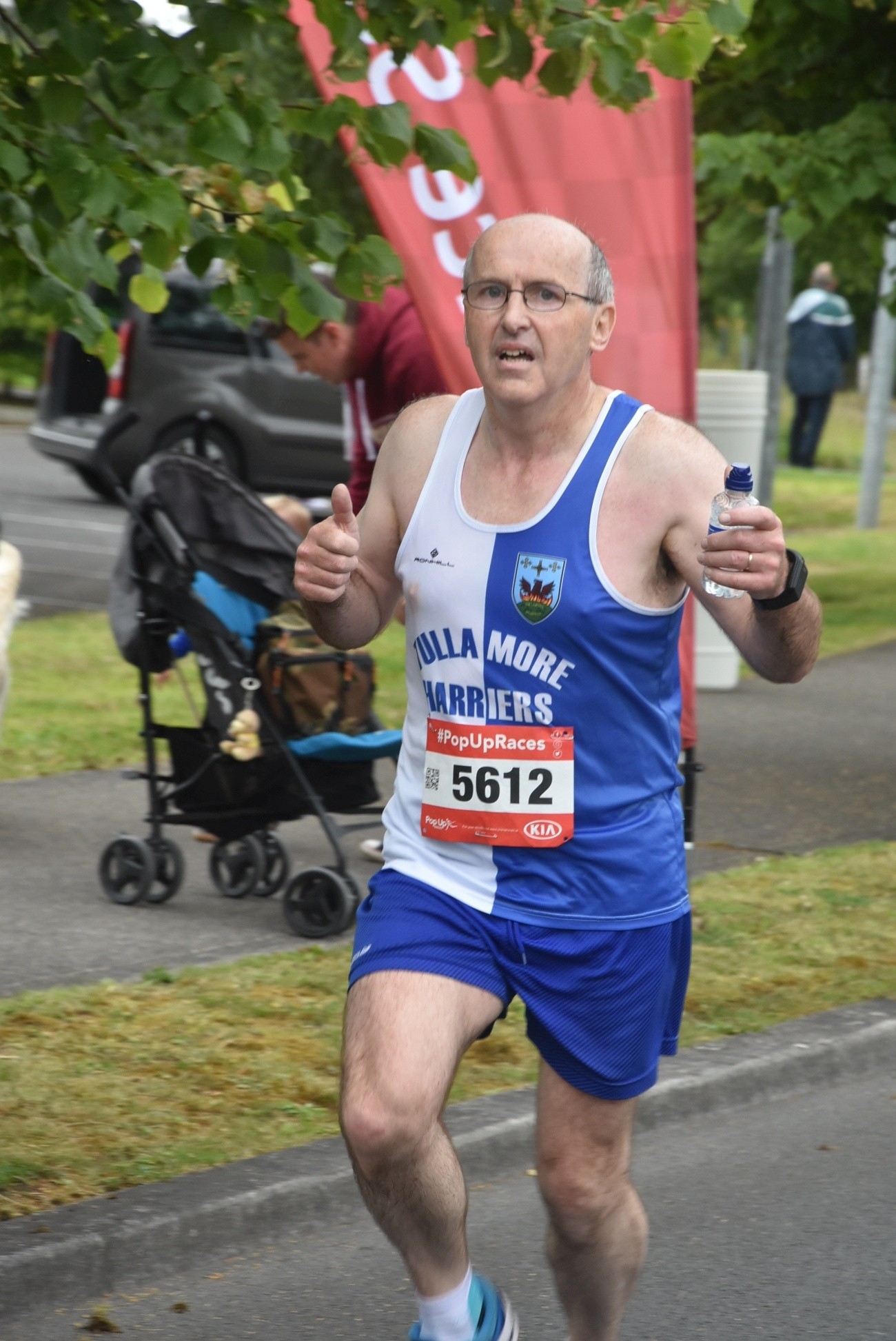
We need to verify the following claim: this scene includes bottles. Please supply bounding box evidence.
[701,461,760,599]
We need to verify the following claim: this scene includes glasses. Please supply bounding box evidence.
[461,280,604,312]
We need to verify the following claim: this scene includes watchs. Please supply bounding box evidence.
[750,548,808,611]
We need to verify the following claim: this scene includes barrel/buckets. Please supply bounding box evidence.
[693,371,764,691]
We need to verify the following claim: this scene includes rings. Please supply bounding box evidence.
[743,551,754,573]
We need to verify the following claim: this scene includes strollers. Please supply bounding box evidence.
[98,449,407,937]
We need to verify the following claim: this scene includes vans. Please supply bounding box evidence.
[23,239,350,506]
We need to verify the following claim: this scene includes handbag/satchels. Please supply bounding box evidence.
[255,613,376,739]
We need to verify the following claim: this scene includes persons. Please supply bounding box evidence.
[784,261,860,466]
[295,214,821,1341]
[155,274,452,862]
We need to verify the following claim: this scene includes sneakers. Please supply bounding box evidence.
[407,1277,519,1341]
